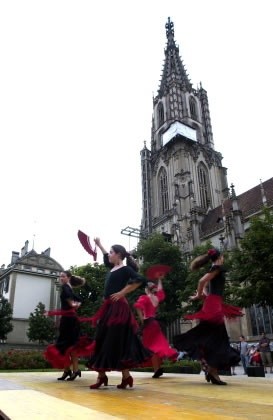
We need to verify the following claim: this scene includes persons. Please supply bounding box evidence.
[171,248,246,385]
[228,332,273,377]
[41,271,92,382]
[85,236,155,390]
[133,272,179,379]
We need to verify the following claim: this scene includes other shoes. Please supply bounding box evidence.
[232,371,237,375]
[270,370,273,374]
[264,370,267,374]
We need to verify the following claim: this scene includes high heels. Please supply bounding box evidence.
[90,375,108,389]
[206,372,227,385]
[152,368,163,378]
[67,369,81,381]
[58,369,72,380]
[117,376,133,388]
